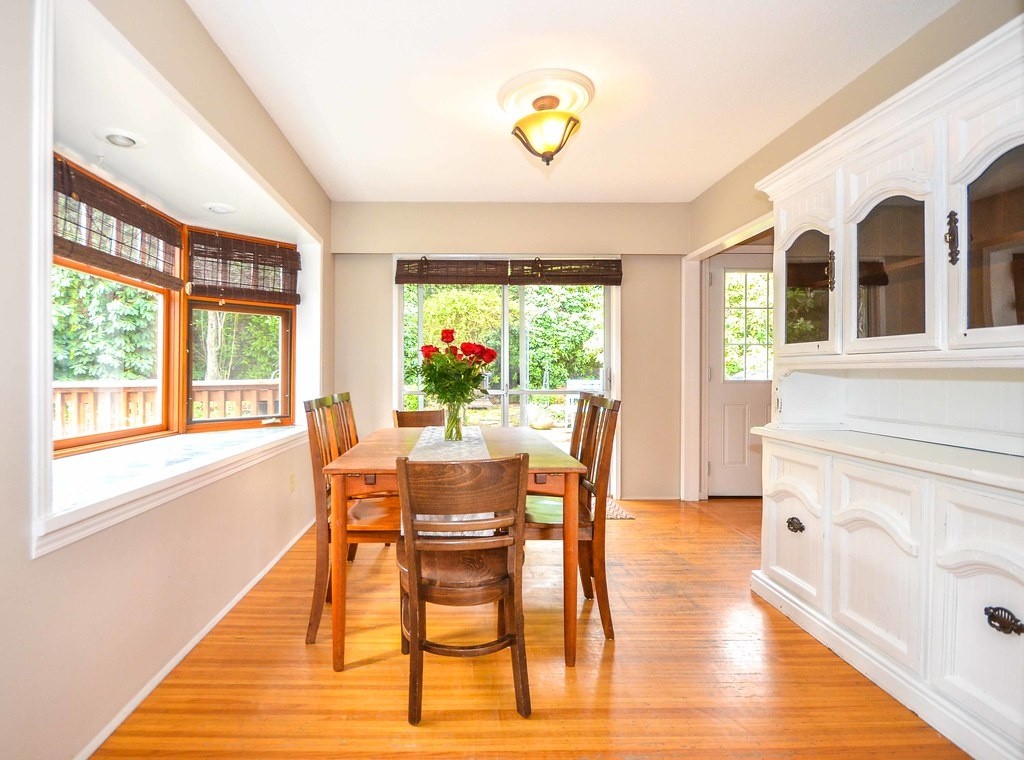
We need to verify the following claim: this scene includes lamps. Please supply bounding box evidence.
[511,94,582,166]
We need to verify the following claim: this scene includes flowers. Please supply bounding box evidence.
[404,329,497,402]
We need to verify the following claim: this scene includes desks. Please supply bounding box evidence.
[321,427,588,671]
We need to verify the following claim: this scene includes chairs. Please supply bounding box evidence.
[395,453,534,724]
[496,397,622,639]
[330,392,400,562]
[570,389,604,578]
[301,396,401,645]
[393,408,445,428]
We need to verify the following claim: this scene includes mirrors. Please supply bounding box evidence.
[856,195,926,338]
[784,228,830,344]
[967,144,1024,331]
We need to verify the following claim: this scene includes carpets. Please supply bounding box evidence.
[590,497,634,520]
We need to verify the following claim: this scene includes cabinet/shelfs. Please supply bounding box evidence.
[950,76,1024,350]
[775,168,842,357]
[842,116,948,355]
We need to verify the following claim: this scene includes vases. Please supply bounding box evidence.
[443,404,464,440]
